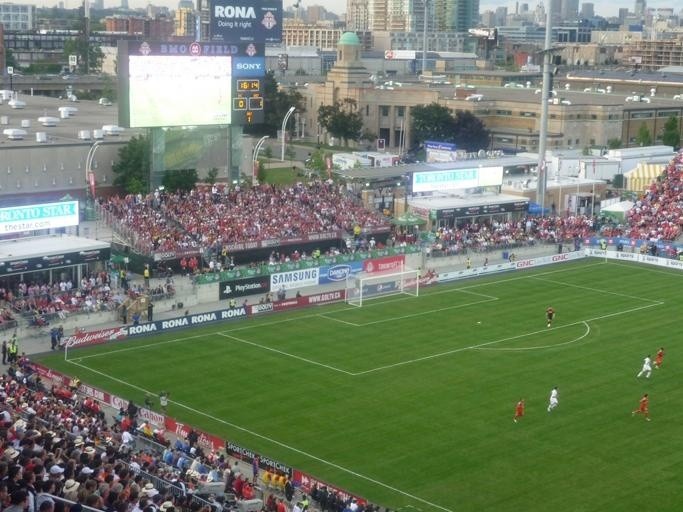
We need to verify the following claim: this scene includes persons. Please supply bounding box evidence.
[546,385,558,412]
[514,397,525,424]
[1,332,382,512]
[623,144,682,261]
[632,392,652,422]
[1,255,176,350]
[546,306,555,328]
[95,172,625,317]
[636,346,664,380]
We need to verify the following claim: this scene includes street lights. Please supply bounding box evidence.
[280,107,295,161]
[86,141,104,184]
[252,135,270,186]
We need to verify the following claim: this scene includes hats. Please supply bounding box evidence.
[4,447,20,459]
[160,500,172,512]
[81,467,95,474]
[50,465,66,475]
[63,478,80,493]
[74,435,96,455]
[141,483,159,498]
